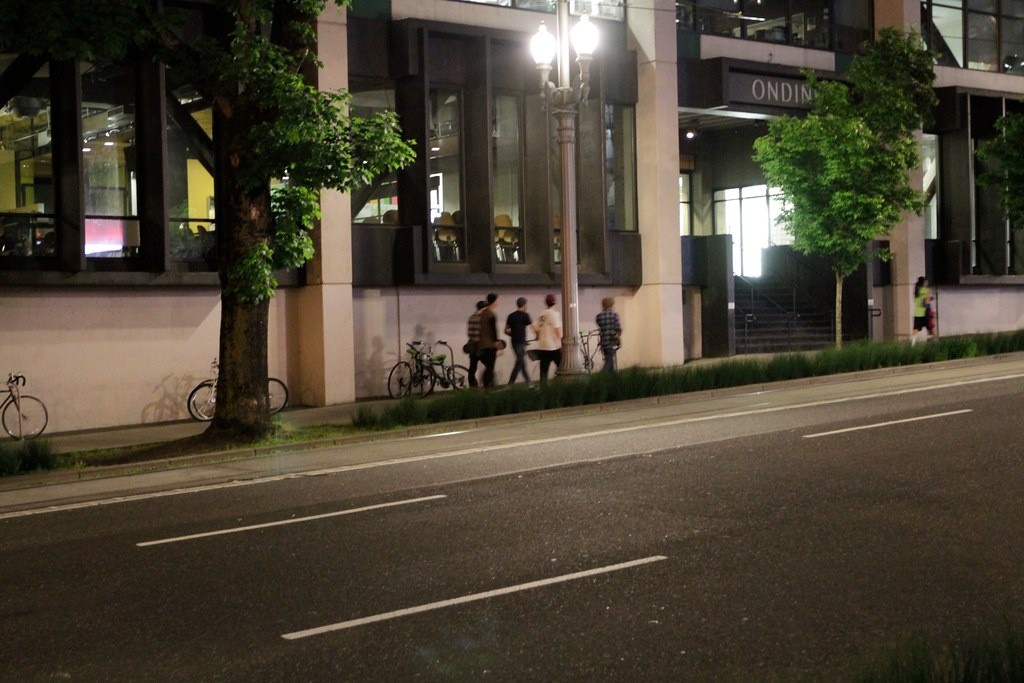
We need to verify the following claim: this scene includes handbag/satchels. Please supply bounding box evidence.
[611,313,621,346]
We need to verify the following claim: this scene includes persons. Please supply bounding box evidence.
[172,225,213,258]
[505,297,537,389]
[595,296,622,372]
[468,301,486,388]
[910,277,935,346]
[479,292,503,391]
[534,294,564,388]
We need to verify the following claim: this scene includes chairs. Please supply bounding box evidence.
[1,203,572,266]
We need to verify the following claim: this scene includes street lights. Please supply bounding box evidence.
[527,12,603,381]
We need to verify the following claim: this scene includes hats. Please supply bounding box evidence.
[517,297,528,305]
[477,301,488,307]
[547,293,557,301]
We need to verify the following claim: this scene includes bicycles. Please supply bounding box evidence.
[531,331,619,386]
[386,338,478,401]
[187,360,289,421]
[0,370,50,440]
